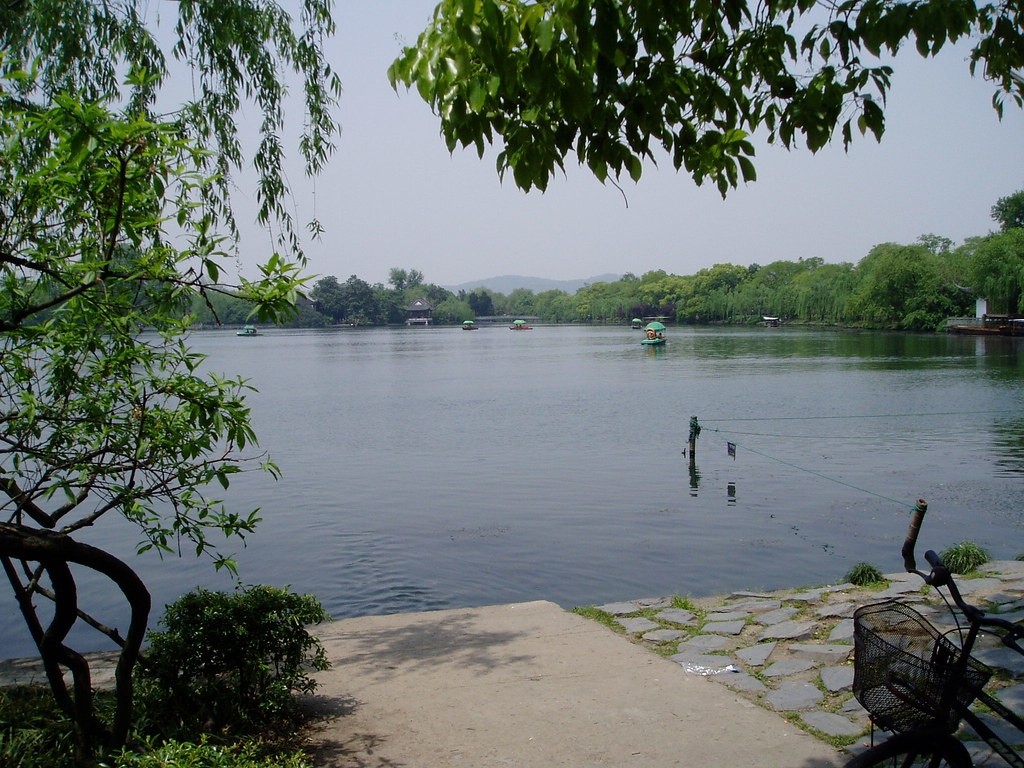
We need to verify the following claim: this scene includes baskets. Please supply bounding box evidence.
[852,600,993,739]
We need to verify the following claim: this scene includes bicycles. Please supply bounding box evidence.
[834,497,1024,768]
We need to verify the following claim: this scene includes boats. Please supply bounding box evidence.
[631,318,643,329]
[949,312,1024,336]
[508,319,533,330]
[641,321,668,346]
[461,320,479,330]
[235,324,265,337]
[762,316,779,328]
